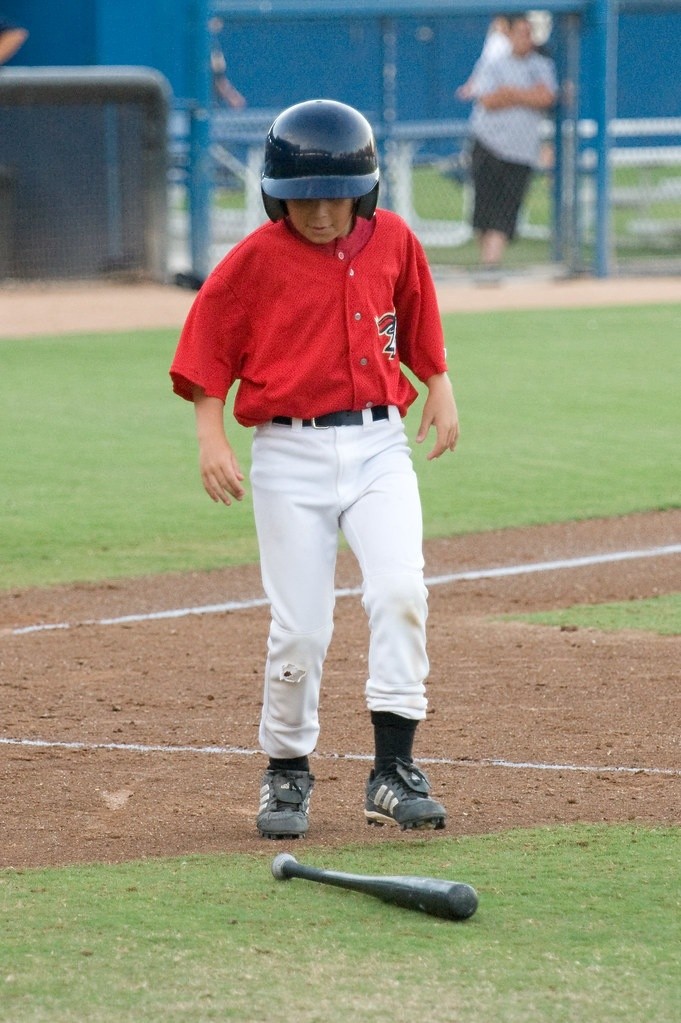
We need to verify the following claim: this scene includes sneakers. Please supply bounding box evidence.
[364,757,446,829]
[257,769,315,840]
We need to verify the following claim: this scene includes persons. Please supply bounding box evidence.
[0,16,31,187]
[168,99,461,848]
[457,11,554,273]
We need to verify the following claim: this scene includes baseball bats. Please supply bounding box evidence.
[270,853,479,921]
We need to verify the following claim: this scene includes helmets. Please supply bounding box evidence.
[260,99,380,224]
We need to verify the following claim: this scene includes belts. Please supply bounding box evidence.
[272,405,389,427]
